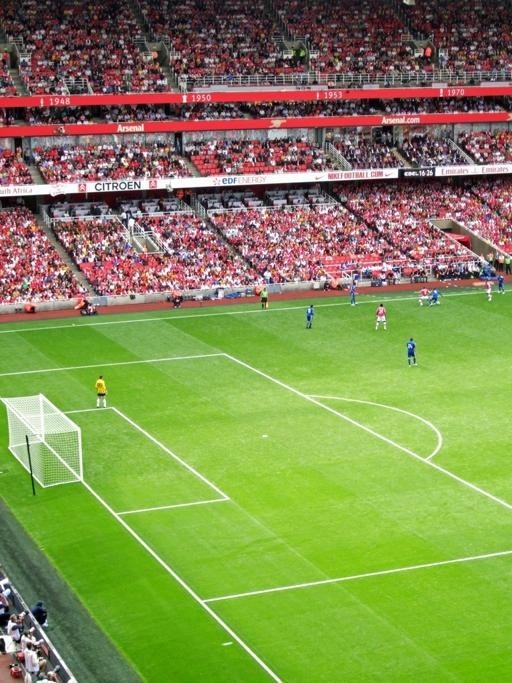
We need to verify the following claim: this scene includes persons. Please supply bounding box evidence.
[406,337,418,367]
[0,578,57,683]
[306,304,315,330]
[95,375,107,407]
[375,303,389,330]
